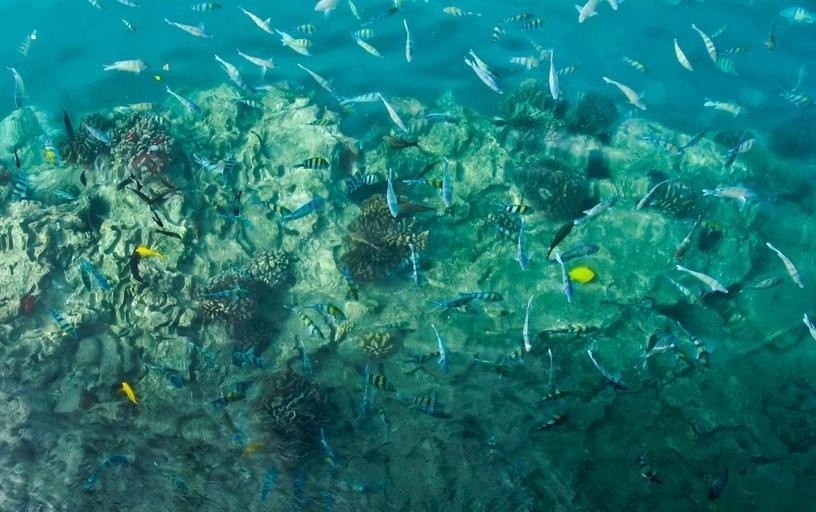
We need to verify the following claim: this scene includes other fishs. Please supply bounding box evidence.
[0,0,816,512]
